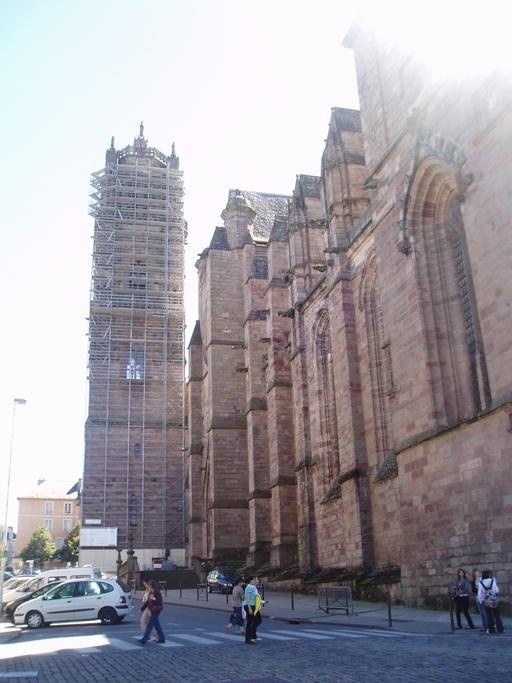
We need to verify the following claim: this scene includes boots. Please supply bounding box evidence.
[239,626,246,634]
[224,623,233,633]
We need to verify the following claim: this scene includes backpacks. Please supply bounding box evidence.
[479,578,497,609]
[148,602,163,613]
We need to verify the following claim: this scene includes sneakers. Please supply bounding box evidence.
[456,626,462,629]
[137,637,146,644]
[480,627,504,634]
[246,637,262,644]
[471,625,475,629]
[156,639,165,643]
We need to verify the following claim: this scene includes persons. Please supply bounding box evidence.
[140,577,159,642]
[137,578,166,644]
[224,576,246,634]
[252,574,267,641]
[126,355,143,379]
[489,570,505,629]
[453,568,475,629]
[7,526,16,540]
[243,575,257,645]
[478,570,506,634]
[471,568,497,632]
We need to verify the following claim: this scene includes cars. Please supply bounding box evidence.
[3,567,134,630]
[207,569,243,593]
